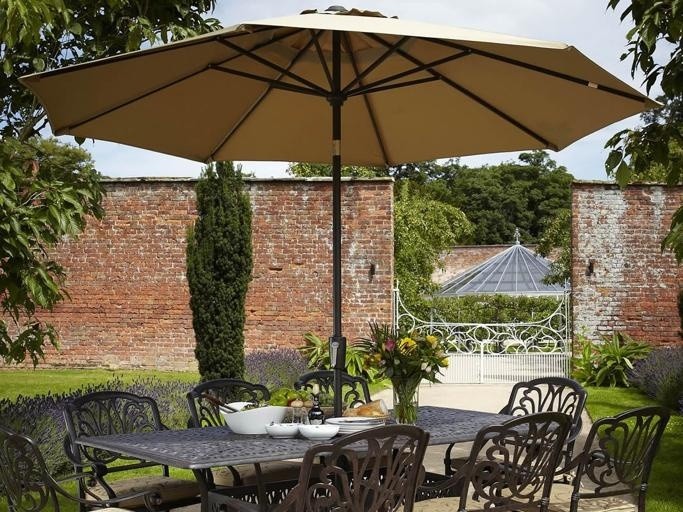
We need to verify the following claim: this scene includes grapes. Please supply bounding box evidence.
[319,393,334,407]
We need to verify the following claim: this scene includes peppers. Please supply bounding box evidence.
[264,387,311,407]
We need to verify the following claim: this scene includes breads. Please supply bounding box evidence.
[342,399,388,417]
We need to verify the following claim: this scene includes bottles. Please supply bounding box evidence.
[308,383,324,425]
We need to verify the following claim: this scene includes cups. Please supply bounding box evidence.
[291,407,309,424]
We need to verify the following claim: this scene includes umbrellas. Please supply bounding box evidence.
[21,9,659,418]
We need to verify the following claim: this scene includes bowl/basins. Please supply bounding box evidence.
[217,401,288,434]
[298,424,340,441]
[265,423,300,439]
[291,406,334,420]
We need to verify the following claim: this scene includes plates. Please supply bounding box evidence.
[324,416,385,435]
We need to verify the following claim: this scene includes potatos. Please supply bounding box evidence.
[291,400,314,408]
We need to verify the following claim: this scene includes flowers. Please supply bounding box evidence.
[348,321,449,422]
